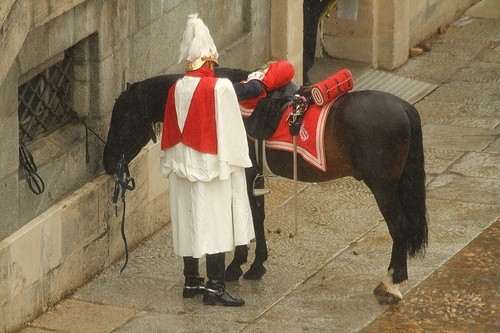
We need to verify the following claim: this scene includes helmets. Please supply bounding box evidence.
[177,12,220,71]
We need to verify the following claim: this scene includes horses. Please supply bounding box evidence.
[101,67,430,303]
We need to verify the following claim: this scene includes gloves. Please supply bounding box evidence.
[247,70,264,81]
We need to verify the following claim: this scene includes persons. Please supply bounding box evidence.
[159,14,268,307]
[303,0,330,86]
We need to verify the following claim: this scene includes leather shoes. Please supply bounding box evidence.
[203,280,246,306]
[182,276,206,298]
